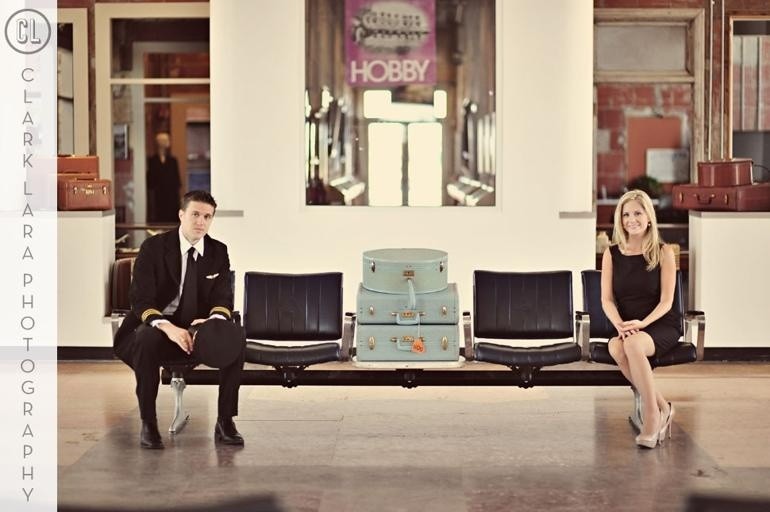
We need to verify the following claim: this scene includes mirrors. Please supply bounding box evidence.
[306,2,497,205]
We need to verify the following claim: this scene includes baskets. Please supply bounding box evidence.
[354,248,461,363]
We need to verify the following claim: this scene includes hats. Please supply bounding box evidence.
[193,318,244,369]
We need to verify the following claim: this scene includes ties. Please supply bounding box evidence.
[180,246,200,319]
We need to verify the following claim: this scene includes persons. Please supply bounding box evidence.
[113,190,245,449]
[146,132,182,225]
[599,187,684,452]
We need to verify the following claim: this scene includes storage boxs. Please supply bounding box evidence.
[646,148,690,184]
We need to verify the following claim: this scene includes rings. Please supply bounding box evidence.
[182,337,186,343]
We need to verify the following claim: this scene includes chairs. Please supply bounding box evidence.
[583,269,707,437]
[232,272,353,389]
[462,271,591,390]
[106,249,238,434]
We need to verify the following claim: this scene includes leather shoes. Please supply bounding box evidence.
[139,424,165,449]
[214,418,244,445]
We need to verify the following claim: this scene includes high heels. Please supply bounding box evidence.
[635,400,676,449]
